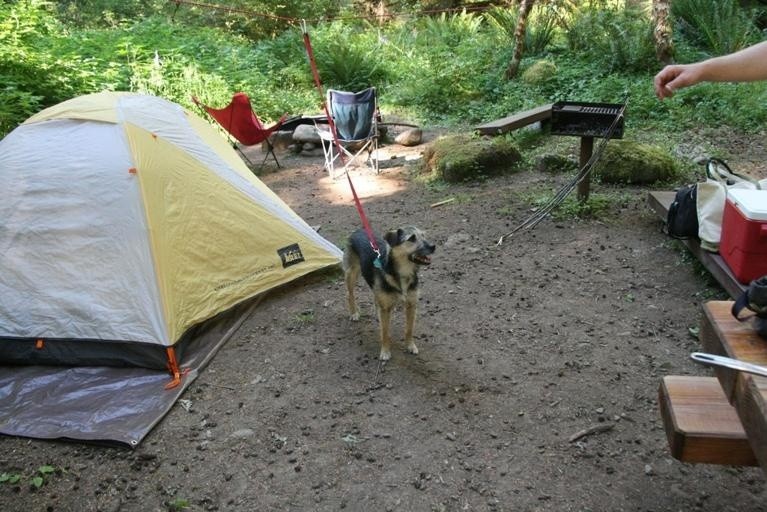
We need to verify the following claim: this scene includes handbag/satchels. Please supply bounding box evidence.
[696,161,767,253]
[667,157,733,237]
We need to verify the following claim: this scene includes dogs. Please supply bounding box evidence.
[342,216,436,362]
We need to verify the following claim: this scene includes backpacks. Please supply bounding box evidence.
[732,275,767,322]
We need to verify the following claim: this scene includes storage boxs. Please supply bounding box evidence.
[717,187,767,284]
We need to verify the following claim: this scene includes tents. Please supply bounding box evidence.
[0,89,344,389]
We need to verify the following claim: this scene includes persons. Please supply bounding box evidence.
[653,41,767,100]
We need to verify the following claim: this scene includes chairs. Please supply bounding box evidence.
[312,84,381,176]
[190,91,290,176]
